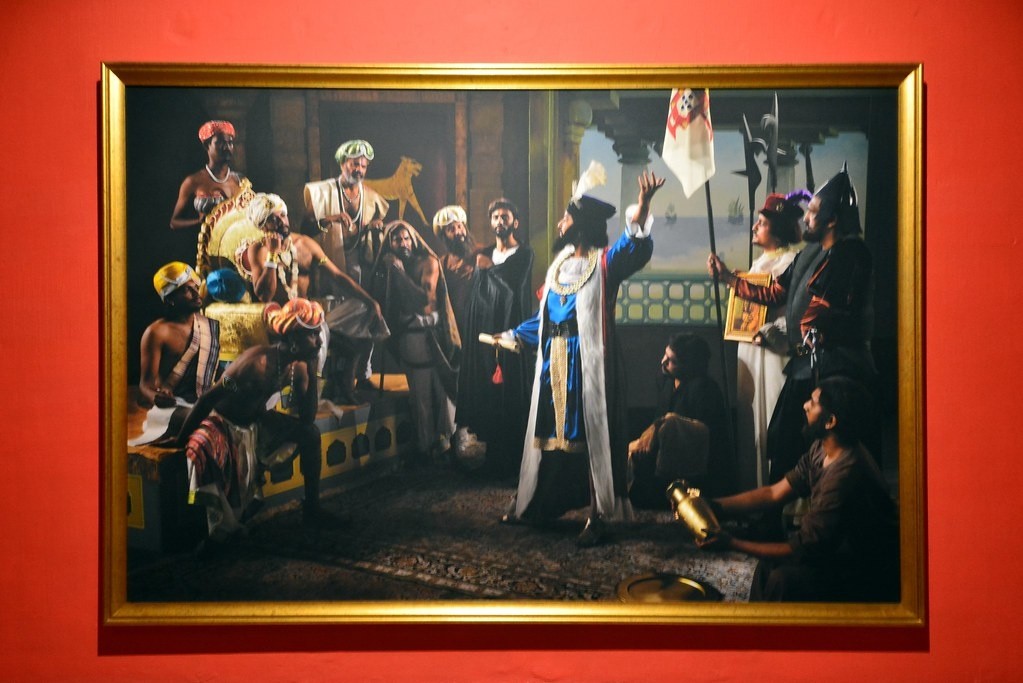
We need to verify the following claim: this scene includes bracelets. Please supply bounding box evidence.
[265,261,278,269]
[265,250,277,263]
[317,216,333,234]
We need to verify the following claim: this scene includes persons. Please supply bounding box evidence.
[477,159,667,545]
[626,159,903,605]
[135,119,536,553]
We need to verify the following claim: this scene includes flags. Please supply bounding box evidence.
[660,88,717,199]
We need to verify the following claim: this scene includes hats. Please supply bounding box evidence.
[759,190,811,221]
[433,205,467,238]
[567,161,616,219]
[273,298,324,334]
[335,140,374,164]
[154,262,201,303]
[249,193,287,229]
[816,160,856,217]
[488,198,519,219]
[198,121,236,144]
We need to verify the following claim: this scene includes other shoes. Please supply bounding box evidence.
[501,512,528,525]
[305,505,338,527]
[576,518,608,547]
[439,439,451,454]
[356,380,379,392]
[321,386,365,405]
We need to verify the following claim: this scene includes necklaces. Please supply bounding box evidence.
[544,243,599,307]
[337,174,364,256]
[205,163,231,183]
[277,339,295,409]
[338,187,362,224]
[341,183,360,203]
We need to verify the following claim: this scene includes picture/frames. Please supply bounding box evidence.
[100,59,926,627]
[303,86,469,258]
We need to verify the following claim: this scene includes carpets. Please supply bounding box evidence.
[250,468,757,603]
